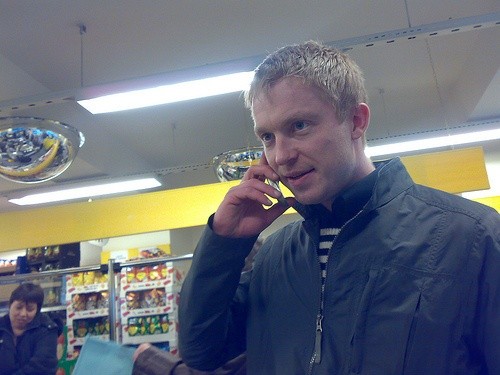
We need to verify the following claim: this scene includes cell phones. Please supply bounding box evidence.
[267,178,287,205]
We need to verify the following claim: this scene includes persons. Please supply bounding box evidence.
[176,39,499,375]
[0,283,58,374]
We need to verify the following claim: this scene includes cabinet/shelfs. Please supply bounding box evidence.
[0,251,192,375]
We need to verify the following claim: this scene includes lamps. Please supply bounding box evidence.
[74,25,263,115]
